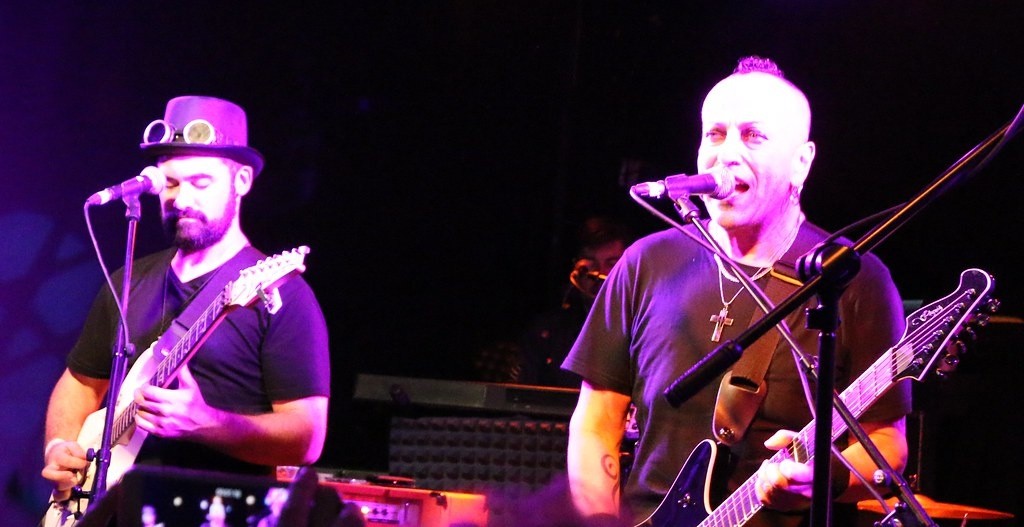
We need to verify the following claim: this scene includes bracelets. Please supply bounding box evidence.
[45,438,65,461]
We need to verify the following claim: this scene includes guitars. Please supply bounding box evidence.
[633,268,1001,527]
[37,244,312,527]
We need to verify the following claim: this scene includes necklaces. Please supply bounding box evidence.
[710,219,800,341]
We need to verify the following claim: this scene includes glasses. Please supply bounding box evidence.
[143,120,215,145]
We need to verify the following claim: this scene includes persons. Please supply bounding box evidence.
[547,220,628,389]
[560,56,915,527]
[39,96,332,527]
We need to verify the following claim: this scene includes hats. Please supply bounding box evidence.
[139,96,264,177]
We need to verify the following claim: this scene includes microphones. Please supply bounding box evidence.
[632,166,736,200]
[85,166,166,205]
